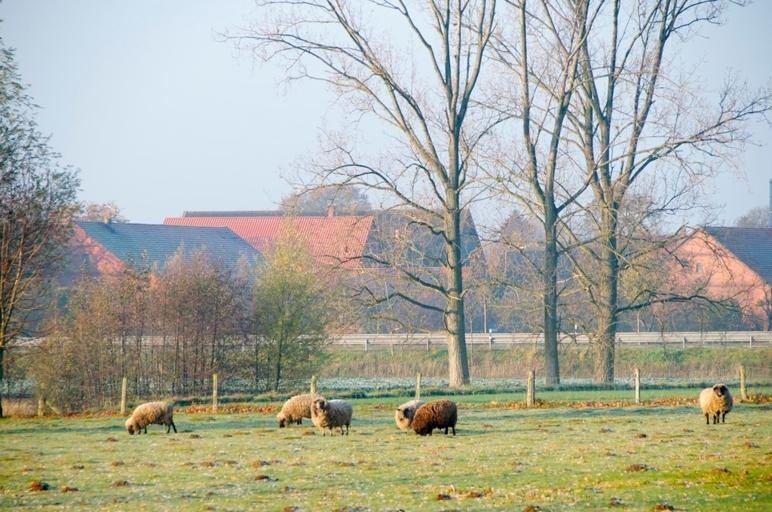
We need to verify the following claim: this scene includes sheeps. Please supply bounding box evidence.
[699,383,733,424]
[125,401,177,435]
[395,399,426,435]
[407,399,458,436]
[310,396,353,437]
[276,393,327,428]
